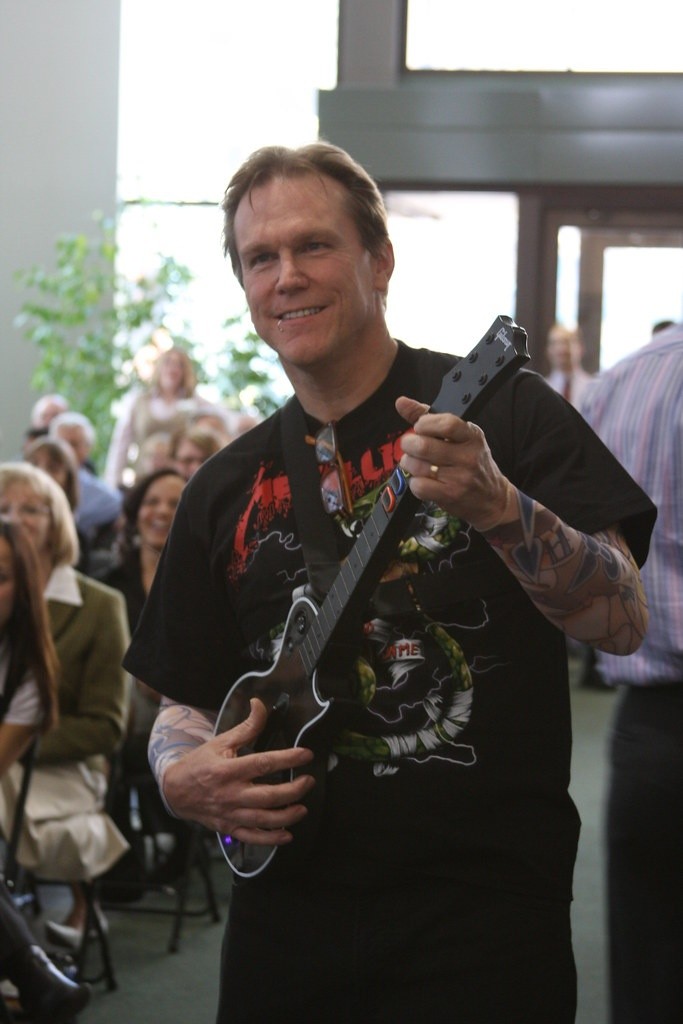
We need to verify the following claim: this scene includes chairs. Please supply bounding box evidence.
[0,672,221,1022]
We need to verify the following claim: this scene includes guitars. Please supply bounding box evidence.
[213,316,531,879]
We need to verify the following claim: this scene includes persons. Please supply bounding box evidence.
[144,142,651,1024]
[0,319,683,1024]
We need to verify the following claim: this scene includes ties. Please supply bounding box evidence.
[564,376,572,403]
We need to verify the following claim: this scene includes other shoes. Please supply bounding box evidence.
[115,830,147,903]
[578,667,616,692]
[44,913,108,951]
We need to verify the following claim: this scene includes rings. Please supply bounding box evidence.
[430,464,439,480]
